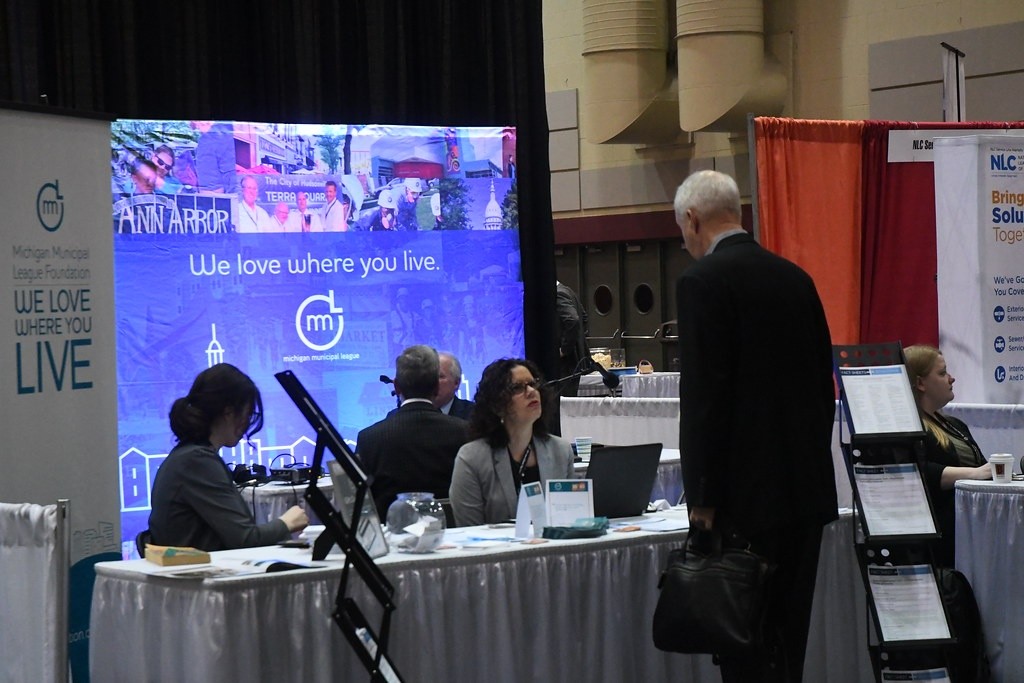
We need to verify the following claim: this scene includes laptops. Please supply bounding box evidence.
[584,442,664,519]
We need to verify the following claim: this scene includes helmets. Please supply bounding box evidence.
[430,193,441,216]
[404,177,423,192]
[377,190,397,209]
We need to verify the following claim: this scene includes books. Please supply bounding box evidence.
[145,544,210,566]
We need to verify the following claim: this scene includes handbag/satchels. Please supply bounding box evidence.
[653,524,773,653]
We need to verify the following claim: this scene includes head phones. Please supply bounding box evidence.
[233,463,269,484]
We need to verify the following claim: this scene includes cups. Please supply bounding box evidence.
[575,436,593,462]
[989,453,1015,484]
[610,348,626,369]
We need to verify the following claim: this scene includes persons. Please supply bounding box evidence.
[555,279,593,369]
[449,358,577,527]
[239,175,345,232]
[391,287,487,363]
[152,144,183,195]
[350,176,444,232]
[902,343,992,570]
[196,123,237,194]
[355,343,487,524]
[131,160,165,194]
[673,170,839,683]
[142,363,309,552]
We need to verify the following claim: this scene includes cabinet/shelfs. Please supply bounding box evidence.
[833,341,961,683]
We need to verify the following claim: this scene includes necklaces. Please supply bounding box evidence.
[324,198,338,220]
[242,201,258,230]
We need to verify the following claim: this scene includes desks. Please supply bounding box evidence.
[954,478,1024,682]
[88,502,879,683]
[579,373,681,397]
[237,449,688,528]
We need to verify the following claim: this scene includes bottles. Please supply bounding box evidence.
[385,492,447,555]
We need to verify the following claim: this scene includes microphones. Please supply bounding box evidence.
[588,357,620,388]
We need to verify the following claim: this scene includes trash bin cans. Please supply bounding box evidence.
[659,319,681,372]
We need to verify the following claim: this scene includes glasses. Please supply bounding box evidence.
[155,154,173,171]
[502,378,539,397]
